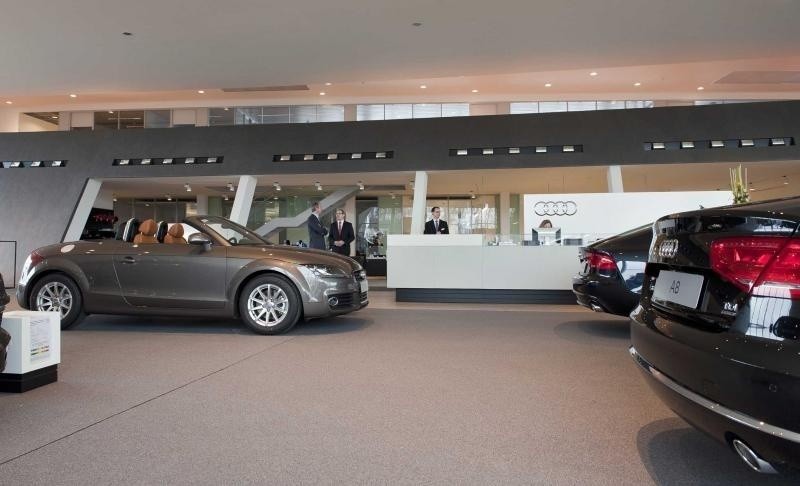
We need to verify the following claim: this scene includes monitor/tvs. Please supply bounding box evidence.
[532,228,562,245]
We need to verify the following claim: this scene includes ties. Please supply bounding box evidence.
[338,222,341,235]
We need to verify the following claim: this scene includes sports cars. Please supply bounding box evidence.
[17,208,372,336]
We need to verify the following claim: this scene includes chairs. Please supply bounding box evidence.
[132,219,187,244]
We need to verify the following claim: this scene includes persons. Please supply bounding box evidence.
[539,220,553,229]
[329,209,356,257]
[308,203,328,250]
[424,206,449,234]
[371,232,384,257]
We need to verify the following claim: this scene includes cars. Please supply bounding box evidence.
[568,198,667,325]
[623,193,800,485]
